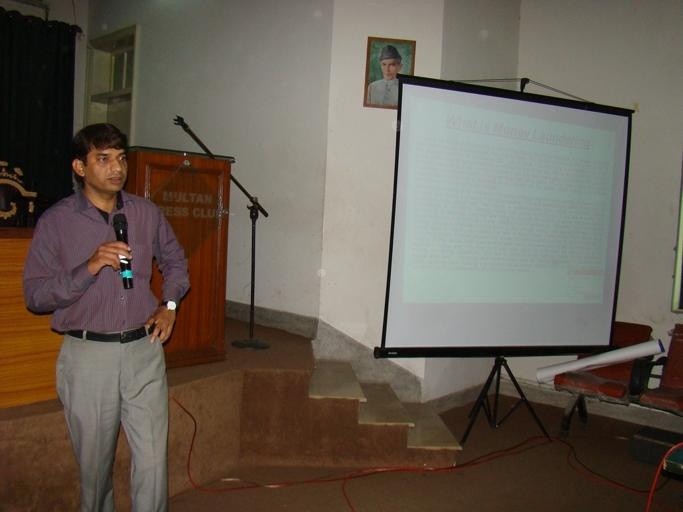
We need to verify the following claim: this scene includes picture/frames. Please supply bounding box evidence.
[362,35,417,108]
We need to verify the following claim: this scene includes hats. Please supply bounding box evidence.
[379,45,402,60]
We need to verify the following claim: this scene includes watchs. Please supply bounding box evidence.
[161,301,178,311]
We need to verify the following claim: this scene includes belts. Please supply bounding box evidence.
[66,321,157,344]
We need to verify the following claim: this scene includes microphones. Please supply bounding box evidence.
[112,212,134,290]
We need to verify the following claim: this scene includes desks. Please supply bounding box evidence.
[124,144,235,370]
[1,220,122,418]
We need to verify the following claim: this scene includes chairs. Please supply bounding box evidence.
[554,322,654,406]
[639,323,683,412]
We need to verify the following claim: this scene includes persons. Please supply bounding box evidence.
[23,123,191,512]
[367,45,403,107]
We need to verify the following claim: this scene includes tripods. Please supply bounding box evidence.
[459,355,551,446]
